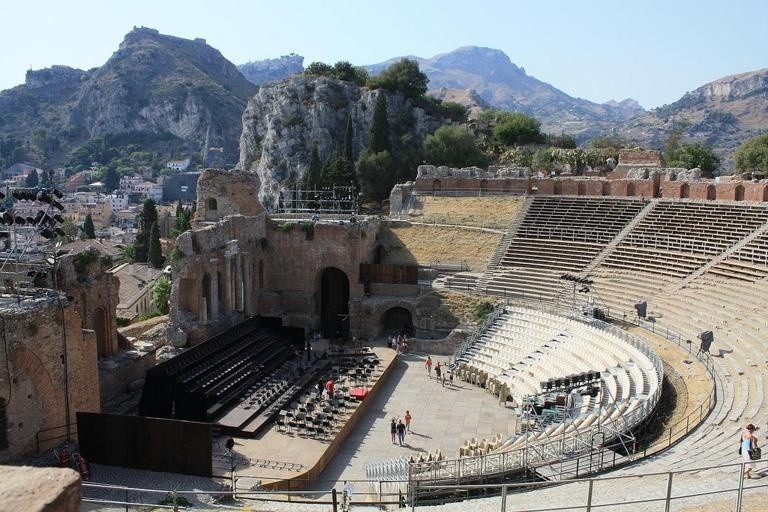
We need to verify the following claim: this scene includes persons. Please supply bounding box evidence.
[738,424,758,479]
[325,378,335,399]
[433,361,455,387]
[397,419,406,447]
[287,343,297,360]
[390,418,396,444]
[318,378,324,397]
[387,333,409,354]
[425,356,432,379]
[307,343,311,361]
[405,410,412,431]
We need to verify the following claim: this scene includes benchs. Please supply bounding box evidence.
[484,194,768,298]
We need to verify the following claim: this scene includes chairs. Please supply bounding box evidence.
[459,433,503,458]
[238,349,381,441]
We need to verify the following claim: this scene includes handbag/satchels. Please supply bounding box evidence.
[738,446,743,455]
[747,447,762,460]
